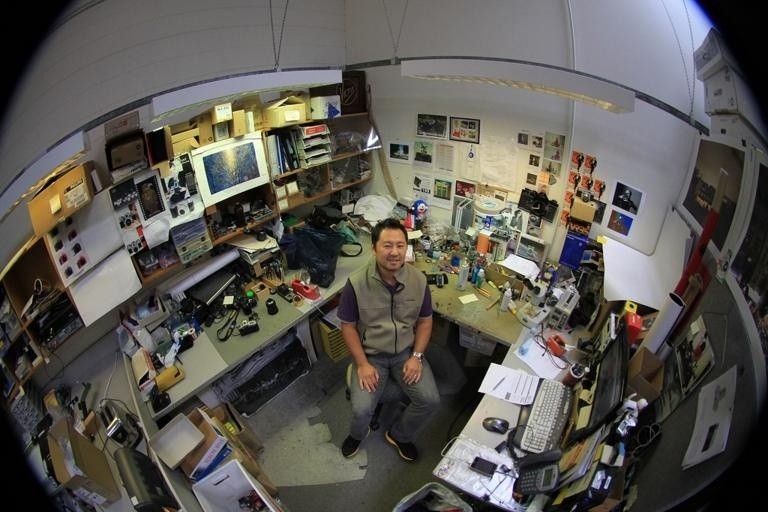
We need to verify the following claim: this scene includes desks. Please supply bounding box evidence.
[399,249,592,512]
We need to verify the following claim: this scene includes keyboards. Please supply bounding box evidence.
[513,377,573,455]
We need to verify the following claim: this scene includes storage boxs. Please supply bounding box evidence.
[693,27,766,153]
[47,412,121,507]
[210,82,341,138]
[146,403,285,512]
[626,346,665,402]
[570,196,597,223]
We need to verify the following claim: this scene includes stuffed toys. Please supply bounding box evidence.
[411,200,429,221]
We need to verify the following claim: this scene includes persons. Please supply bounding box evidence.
[336,219,441,460]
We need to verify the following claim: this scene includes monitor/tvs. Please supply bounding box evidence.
[585,328,629,433]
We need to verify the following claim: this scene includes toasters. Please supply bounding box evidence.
[98,401,142,451]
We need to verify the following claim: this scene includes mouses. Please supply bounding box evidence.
[483,417,509,434]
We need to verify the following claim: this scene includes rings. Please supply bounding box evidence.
[414,375,417,377]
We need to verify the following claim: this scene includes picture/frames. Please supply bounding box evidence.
[449,116,480,144]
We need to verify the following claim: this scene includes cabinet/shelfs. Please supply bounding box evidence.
[0,113,373,414]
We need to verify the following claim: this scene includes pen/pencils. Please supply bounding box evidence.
[493,378,505,390]
[477,287,501,309]
[574,359,586,375]
[508,300,516,315]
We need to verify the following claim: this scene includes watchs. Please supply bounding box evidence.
[412,351,424,360]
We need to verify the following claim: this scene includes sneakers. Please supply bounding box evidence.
[341,425,371,459]
[385,429,419,462]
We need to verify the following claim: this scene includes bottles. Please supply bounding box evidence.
[457,260,469,290]
[501,288,514,312]
[265,298,278,314]
[235,202,244,227]
[519,328,535,355]
[475,269,486,288]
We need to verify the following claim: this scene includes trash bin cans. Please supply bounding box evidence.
[393,482,473,512]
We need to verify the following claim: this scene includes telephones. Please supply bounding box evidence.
[516,449,562,494]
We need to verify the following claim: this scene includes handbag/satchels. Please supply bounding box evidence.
[291,222,347,291]
[305,203,349,230]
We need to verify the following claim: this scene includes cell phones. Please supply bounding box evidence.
[470,456,497,477]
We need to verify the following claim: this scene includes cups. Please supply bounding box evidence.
[563,366,584,387]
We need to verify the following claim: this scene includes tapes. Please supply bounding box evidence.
[300,281,306,287]
